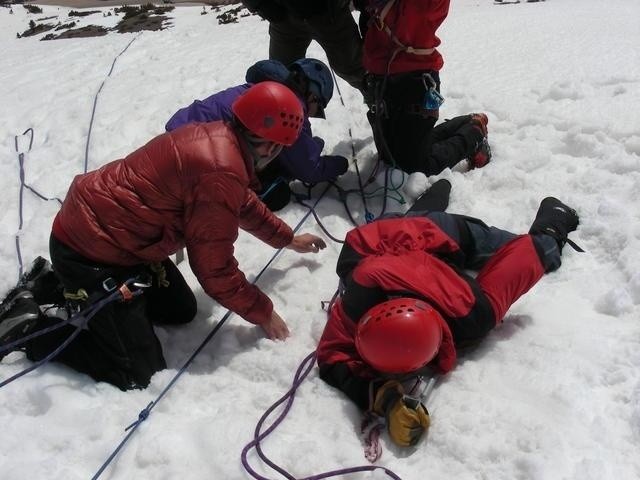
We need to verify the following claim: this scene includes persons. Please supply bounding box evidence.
[0,80,329,393]
[164,56,350,214]
[348,1,493,177]
[312,173,582,448]
[241,0,372,114]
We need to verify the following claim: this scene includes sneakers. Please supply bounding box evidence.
[0,255,65,364]
[466,112,492,172]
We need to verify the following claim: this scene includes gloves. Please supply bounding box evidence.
[372,379,431,449]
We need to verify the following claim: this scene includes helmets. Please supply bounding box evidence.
[289,57,335,109]
[229,80,306,148]
[353,295,445,376]
